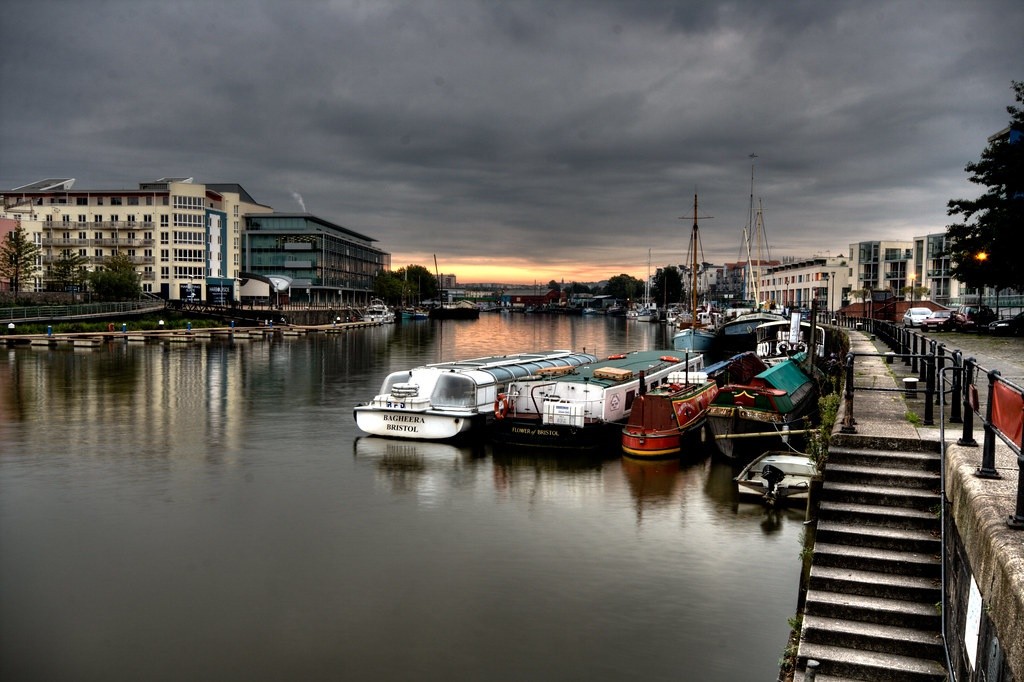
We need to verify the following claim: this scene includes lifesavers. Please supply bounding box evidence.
[747,326,752,334]
[756,343,769,356]
[607,355,627,360]
[494,393,509,420]
[660,356,680,362]
[776,341,791,354]
[793,341,808,354]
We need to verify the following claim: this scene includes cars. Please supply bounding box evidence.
[902,303,1024,337]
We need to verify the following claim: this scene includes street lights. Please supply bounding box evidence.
[831,271,836,319]
[910,274,915,307]
[976,252,985,336]
[785,280,789,307]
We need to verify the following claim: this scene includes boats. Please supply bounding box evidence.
[400,301,430,319]
[621,351,769,457]
[353,349,598,440]
[582,302,625,316]
[706,351,825,460]
[365,298,396,323]
[737,448,821,507]
[487,349,706,453]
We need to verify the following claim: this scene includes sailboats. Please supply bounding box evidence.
[626,247,660,322]
[667,151,824,361]
